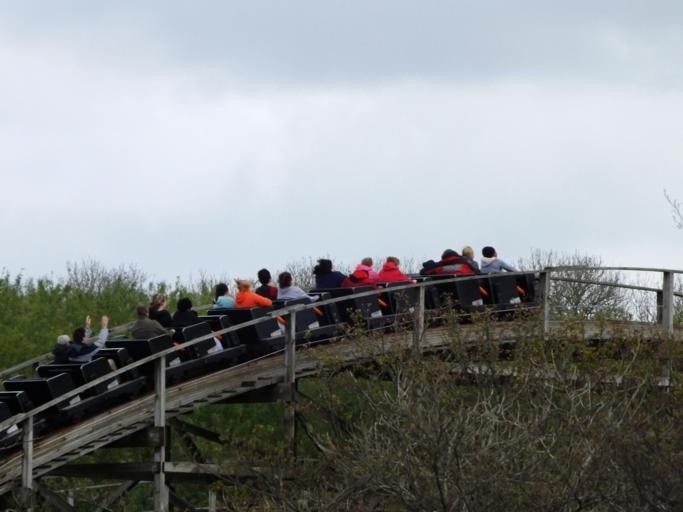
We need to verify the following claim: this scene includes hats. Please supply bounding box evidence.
[57,334,71,344]
[482,247,494,257]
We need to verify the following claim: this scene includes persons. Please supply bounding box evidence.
[423,248,477,276]
[211,282,235,307]
[420,259,438,275]
[57,313,92,347]
[274,272,319,304]
[254,268,278,300]
[377,256,416,283]
[69,316,109,363]
[340,267,382,298]
[149,293,170,326]
[478,247,516,273]
[462,245,479,274]
[132,306,176,351]
[354,258,378,282]
[233,277,272,311]
[313,260,346,287]
[166,296,201,348]
